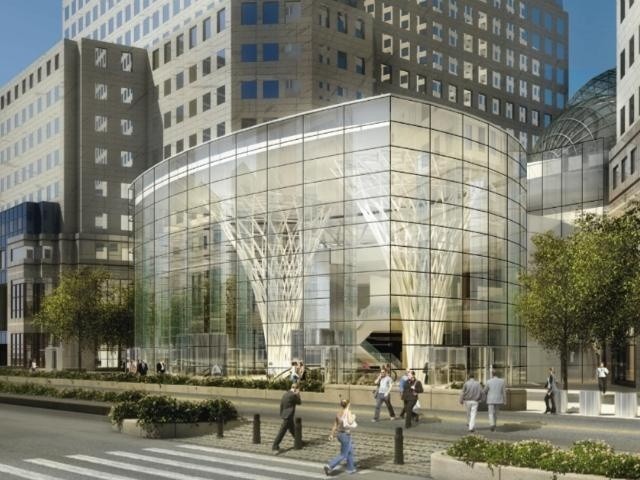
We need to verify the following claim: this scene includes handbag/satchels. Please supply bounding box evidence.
[343,416,357,428]
[373,389,379,398]
